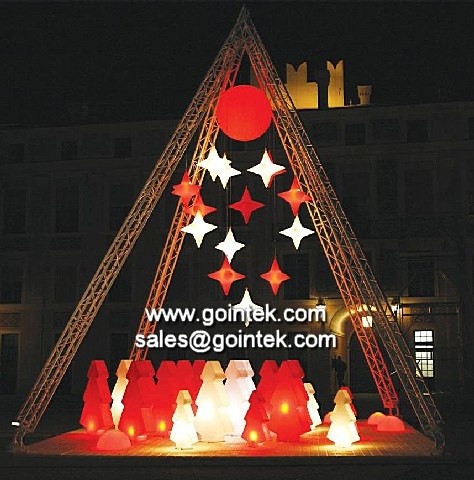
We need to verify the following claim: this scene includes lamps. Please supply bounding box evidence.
[77,59,414,447]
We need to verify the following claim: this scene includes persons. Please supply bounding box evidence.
[335,355,346,388]
